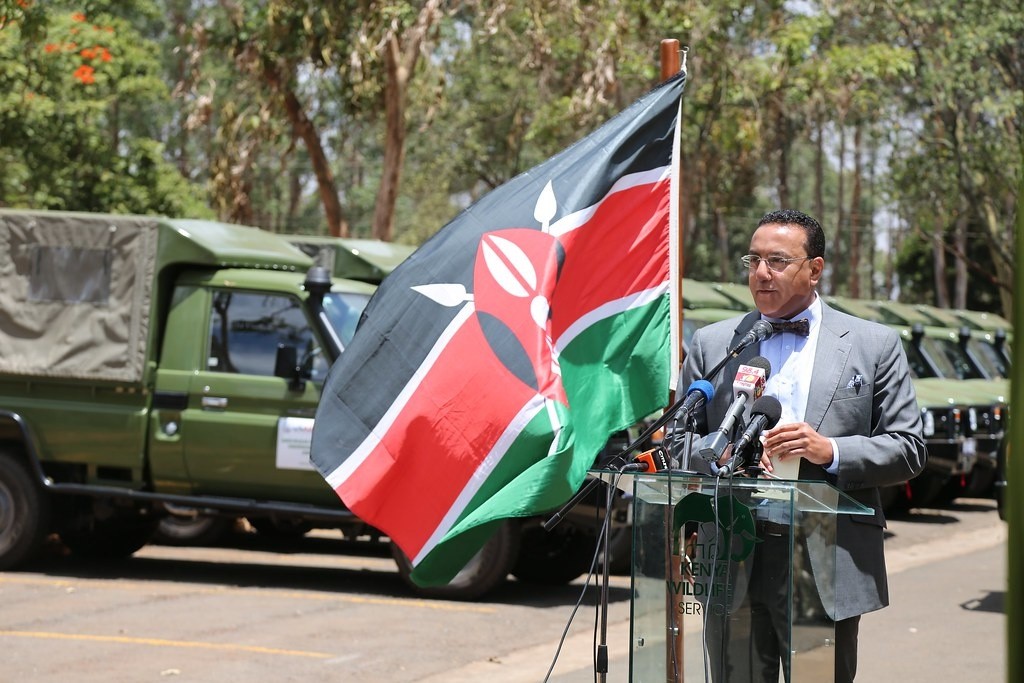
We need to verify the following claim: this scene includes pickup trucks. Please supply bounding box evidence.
[0,208,1010,576]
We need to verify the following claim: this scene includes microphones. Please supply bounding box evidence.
[619,446,680,474]
[733,320,774,351]
[674,357,782,477]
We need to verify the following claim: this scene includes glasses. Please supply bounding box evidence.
[740,255,813,269]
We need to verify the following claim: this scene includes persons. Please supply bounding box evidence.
[662,209,930,683]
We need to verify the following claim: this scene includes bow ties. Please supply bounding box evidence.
[765,317,809,336]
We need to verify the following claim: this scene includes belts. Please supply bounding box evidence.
[755,520,804,539]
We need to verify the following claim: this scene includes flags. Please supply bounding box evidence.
[309,69,687,590]
